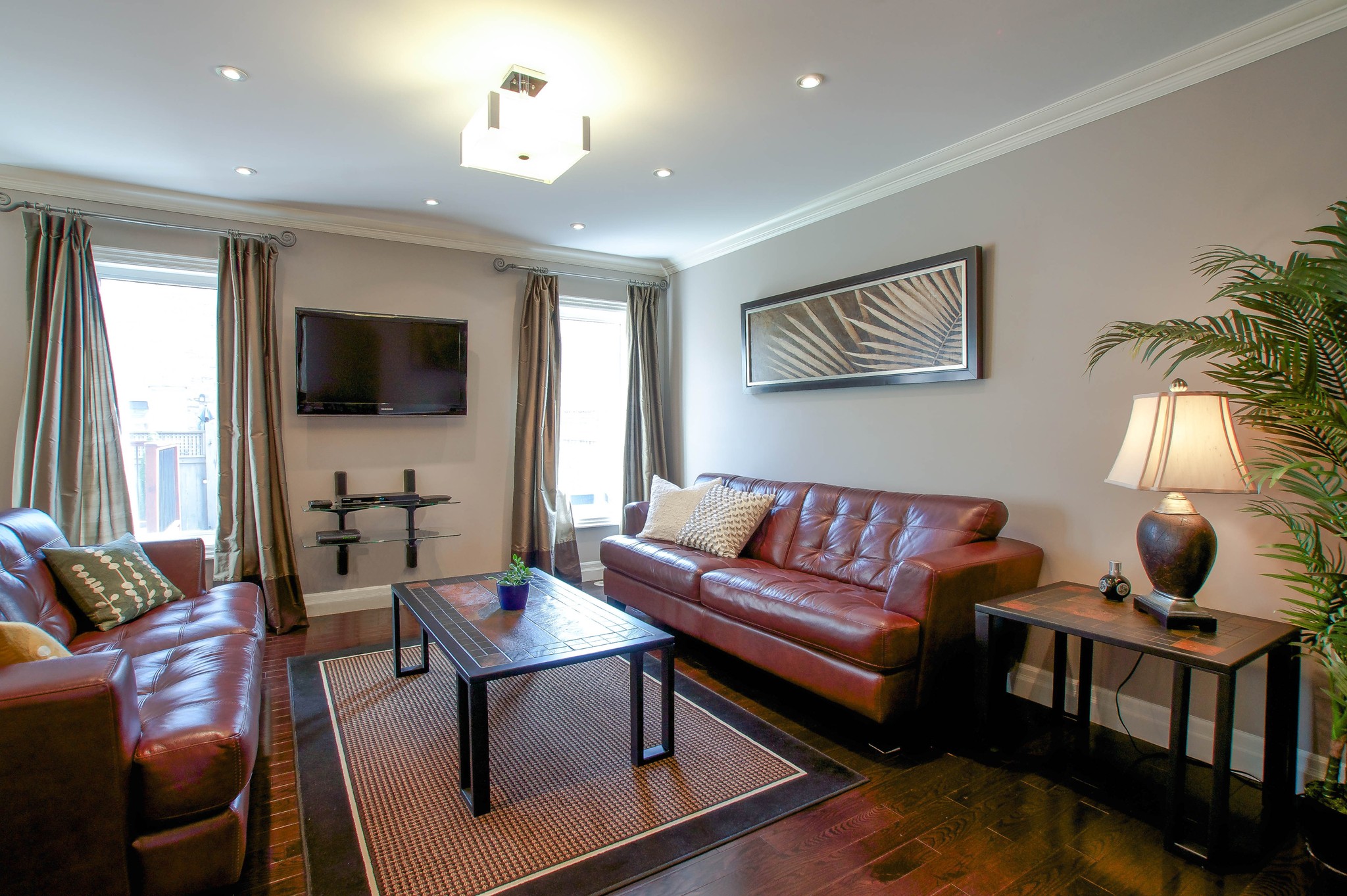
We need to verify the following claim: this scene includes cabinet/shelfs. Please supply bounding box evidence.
[300,470,464,575]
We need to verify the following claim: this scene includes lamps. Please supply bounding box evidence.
[459,62,591,183]
[1101,375,1260,636]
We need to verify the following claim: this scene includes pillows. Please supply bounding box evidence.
[37,533,187,632]
[637,473,722,542]
[673,486,777,559]
[0,621,73,668]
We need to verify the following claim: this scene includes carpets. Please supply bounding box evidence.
[285,635,872,896]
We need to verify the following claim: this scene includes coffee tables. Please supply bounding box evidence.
[391,567,676,817]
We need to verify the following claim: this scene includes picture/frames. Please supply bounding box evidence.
[739,244,985,395]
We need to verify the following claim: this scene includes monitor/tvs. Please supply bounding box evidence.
[295,307,469,418]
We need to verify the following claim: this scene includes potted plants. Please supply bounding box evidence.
[1084,193,1347,896]
[487,554,534,612]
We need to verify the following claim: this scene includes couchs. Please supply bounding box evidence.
[0,507,265,896]
[599,473,1045,768]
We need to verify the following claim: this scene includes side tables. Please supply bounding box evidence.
[972,577,1305,872]
